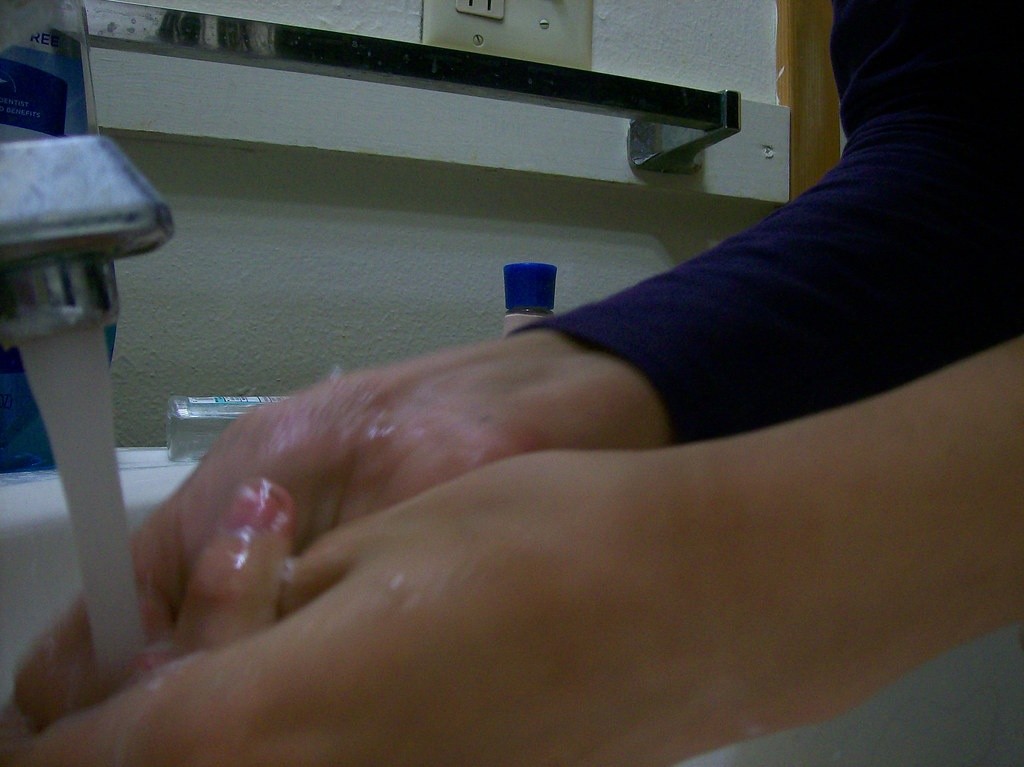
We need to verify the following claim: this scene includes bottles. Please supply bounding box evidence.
[501,261,559,336]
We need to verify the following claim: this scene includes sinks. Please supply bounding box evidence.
[0,448,211,695]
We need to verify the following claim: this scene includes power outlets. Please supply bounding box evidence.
[420,0,595,70]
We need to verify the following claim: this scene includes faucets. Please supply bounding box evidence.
[0,128,176,351]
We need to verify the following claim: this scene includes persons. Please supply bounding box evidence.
[0,0,1024,767]
